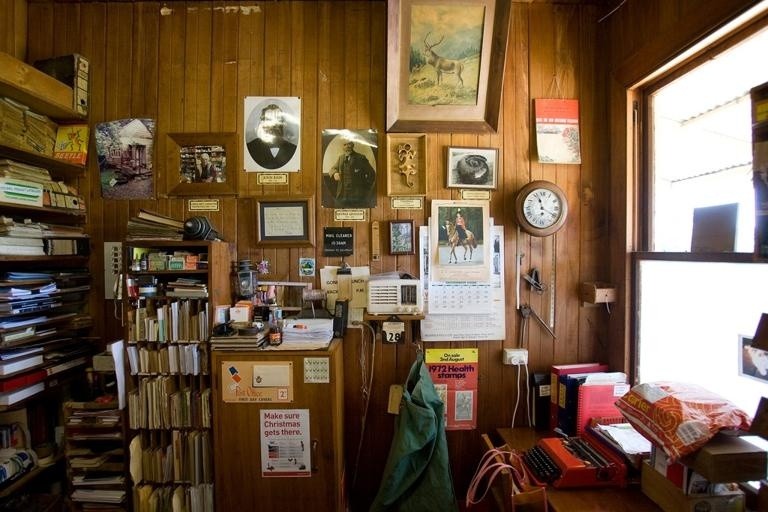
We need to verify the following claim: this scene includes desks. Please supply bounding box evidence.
[493,424,666,511]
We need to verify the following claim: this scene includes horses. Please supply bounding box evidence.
[442,220,477,264]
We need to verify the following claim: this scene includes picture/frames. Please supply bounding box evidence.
[164,132,239,197]
[383,0,511,134]
[387,218,416,255]
[253,195,318,249]
[385,133,428,198]
[444,145,499,190]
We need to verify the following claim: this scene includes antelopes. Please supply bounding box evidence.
[422,32,465,88]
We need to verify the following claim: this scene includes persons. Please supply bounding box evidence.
[455,211,467,244]
[247,105,297,169]
[195,153,216,182]
[329,138,374,201]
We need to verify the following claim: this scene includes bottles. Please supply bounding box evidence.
[270,319,282,346]
[140,253,147,271]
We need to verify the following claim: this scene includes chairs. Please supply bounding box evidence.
[478,432,550,512]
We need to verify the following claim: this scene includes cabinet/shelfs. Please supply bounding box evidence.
[0,50,92,497]
[212,339,348,510]
[120,237,232,511]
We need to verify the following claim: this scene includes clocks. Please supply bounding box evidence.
[513,178,568,239]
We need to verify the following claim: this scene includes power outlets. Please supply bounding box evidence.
[503,347,528,366]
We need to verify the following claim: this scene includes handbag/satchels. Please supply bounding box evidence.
[492,445,549,512]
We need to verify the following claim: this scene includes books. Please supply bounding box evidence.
[0,161,96,450]
[210,328,269,350]
[126,209,186,240]
[66,410,127,510]
[129,278,213,511]
